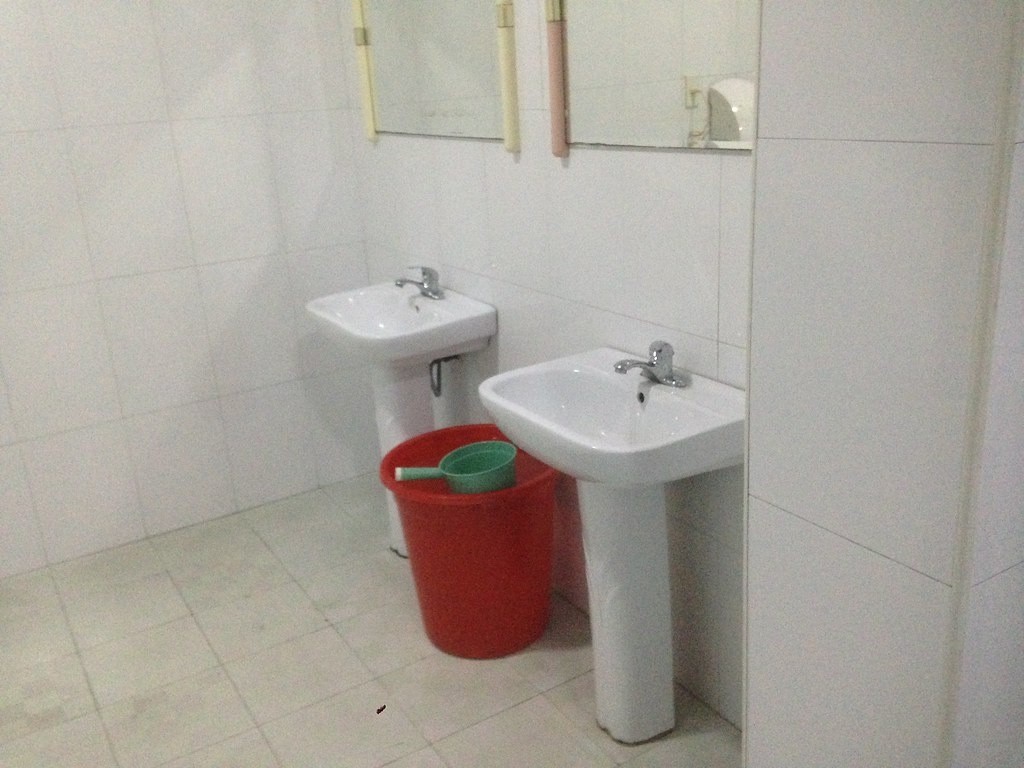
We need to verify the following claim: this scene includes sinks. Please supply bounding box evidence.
[306,278,496,368]
[478,345,746,485]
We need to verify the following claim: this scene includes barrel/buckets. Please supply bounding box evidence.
[379,424,557,660]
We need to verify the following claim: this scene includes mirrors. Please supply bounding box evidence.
[544,0,761,156]
[350,0,522,152]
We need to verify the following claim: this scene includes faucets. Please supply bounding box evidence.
[613,339,687,389]
[396,265,445,300]
